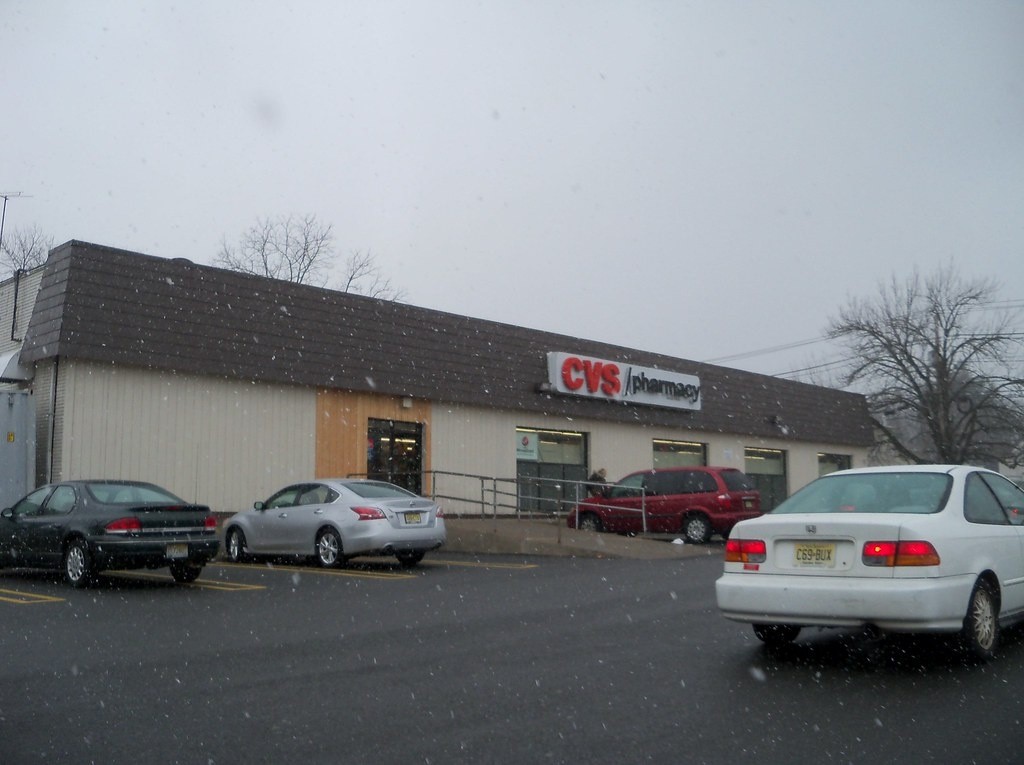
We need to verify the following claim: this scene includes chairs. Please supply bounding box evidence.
[909,487,937,511]
[840,484,885,512]
[301,491,319,505]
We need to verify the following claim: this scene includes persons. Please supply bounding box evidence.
[585,468,608,496]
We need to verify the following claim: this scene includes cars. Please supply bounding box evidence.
[566,466,764,546]
[0,479,222,589]
[222,477,448,570]
[714,463,1024,665]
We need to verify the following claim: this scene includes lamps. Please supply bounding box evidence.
[767,416,783,425]
[536,381,553,392]
